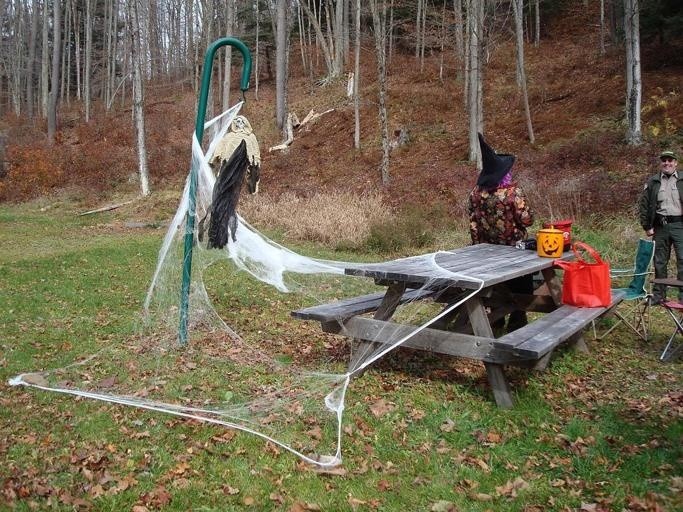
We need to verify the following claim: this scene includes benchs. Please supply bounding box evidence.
[492,291,626,372]
[289,288,435,343]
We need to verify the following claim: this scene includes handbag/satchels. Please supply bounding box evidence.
[552,242,613,309]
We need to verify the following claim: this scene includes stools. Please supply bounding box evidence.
[656,299,682,363]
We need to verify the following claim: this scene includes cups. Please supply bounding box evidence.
[540,220,571,251]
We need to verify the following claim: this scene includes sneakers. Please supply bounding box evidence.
[643,296,668,305]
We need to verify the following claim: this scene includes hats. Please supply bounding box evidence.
[475,131,516,187]
[659,150,679,160]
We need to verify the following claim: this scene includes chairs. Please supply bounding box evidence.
[589,237,656,343]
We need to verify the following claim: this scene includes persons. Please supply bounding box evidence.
[638,151,683,305]
[467,168,535,331]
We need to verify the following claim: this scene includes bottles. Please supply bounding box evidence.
[534,224,565,260]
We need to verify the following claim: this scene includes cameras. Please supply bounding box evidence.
[516,238,537,250]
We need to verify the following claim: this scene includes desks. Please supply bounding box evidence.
[342,242,590,416]
